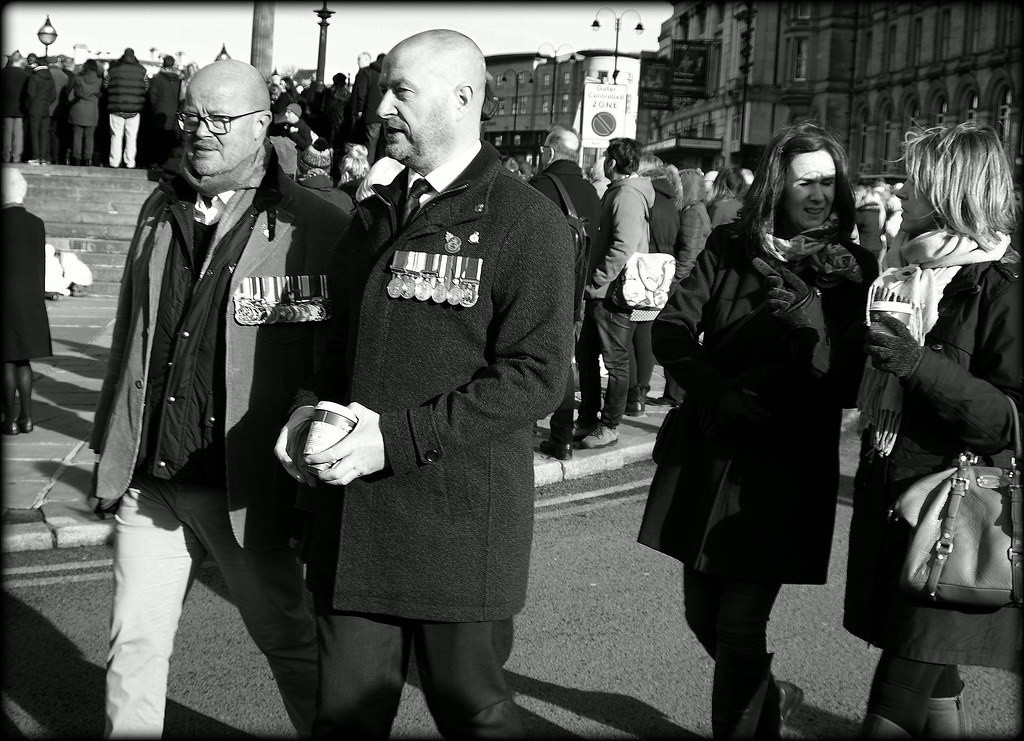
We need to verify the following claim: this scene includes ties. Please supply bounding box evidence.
[397,178,430,229]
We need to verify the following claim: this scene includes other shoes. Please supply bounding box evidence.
[28,158,98,166]
[648,397,674,406]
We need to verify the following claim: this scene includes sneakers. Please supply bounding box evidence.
[582,425,618,448]
[572,417,601,438]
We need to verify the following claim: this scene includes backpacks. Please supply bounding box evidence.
[540,172,592,322]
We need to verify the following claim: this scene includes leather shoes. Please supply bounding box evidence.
[0,422,18,434]
[540,440,572,460]
[624,401,642,416]
[16,418,33,431]
[775,678,803,730]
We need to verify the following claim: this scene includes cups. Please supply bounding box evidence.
[304,401,358,477]
[869,301,914,371]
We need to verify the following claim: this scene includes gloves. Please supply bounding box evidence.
[864,312,922,378]
[753,256,809,314]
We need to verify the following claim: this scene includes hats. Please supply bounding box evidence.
[287,103,302,117]
[298,137,331,175]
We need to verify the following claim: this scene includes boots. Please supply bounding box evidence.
[859,714,913,741]
[926,679,973,741]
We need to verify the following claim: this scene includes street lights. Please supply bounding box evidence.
[502,68,534,155]
[592,7,645,85]
[535,42,577,128]
[37,14,58,57]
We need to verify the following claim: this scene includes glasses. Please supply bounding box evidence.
[540,145,555,154]
[175,110,265,135]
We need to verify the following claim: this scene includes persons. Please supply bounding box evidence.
[0,166,54,436]
[753,117,1024,741]
[265,51,389,223]
[91,59,354,741]
[525,127,757,462]
[272,30,574,741]
[0,48,200,170]
[637,122,879,741]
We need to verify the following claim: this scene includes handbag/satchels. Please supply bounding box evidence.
[894,395,1024,605]
[603,251,675,322]
[65,75,84,105]
